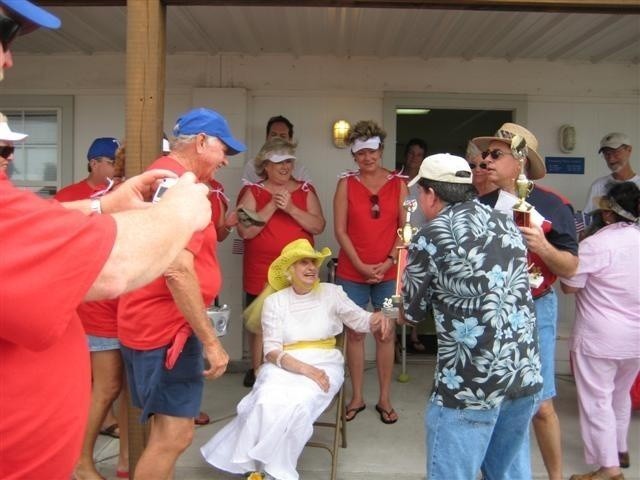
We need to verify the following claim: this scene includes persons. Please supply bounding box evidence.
[53,116,639,478]
[0,0,215,480]
[0,111,30,182]
[113,105,250,480]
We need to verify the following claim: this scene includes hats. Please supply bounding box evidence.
[172,108,246,155]
[1,122,28,141]
[407,153,473,187]
[263,150,296,163]
[472,122,546,179]
[352,136,381,152]
[1,0,61,30]
[268,238,331,291]
[592,195,639,222]
[598,132,632,153]
[87,137,120,159]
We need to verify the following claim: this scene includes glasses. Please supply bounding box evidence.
[0,146,14,159]
[371,195,380,218]
[482,151,513,160]
[470,164,488,169]
[0,16,19,51]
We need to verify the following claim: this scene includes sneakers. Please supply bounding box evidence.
[619,452,630,468]
[570,466,624,479]
[195,412,209,425]
[244,369,256,387]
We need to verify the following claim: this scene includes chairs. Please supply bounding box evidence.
[304,322,349,479]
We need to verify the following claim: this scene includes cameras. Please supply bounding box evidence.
[206,304,230,337]
[152,178,179,204]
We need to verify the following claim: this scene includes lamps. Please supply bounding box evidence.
[558,123,577,153]
[333,119,351,149]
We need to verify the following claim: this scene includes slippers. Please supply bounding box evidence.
[376,404,398,424]
[410,340,427,353]
[341,402,365,422]
[99,424,120,438]
